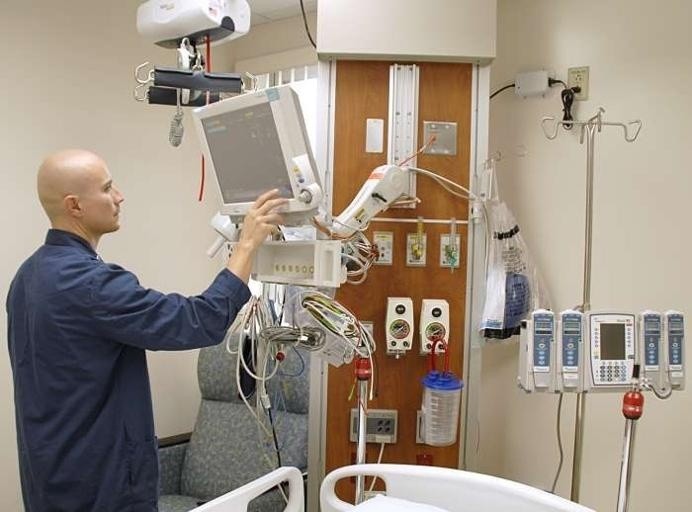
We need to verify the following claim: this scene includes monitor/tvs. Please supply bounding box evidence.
[191,85,323,224]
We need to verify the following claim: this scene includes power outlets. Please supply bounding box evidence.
[347,406,398,447]
[565,65,590,103]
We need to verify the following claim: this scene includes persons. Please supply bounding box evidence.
[6,148,289,512]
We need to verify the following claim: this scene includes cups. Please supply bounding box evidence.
[419,367,465,449]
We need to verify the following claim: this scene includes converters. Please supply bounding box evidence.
[514,70,550,97]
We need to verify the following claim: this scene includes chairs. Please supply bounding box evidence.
[156,328,318,512]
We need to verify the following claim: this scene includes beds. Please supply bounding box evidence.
[184,462,599,512]
